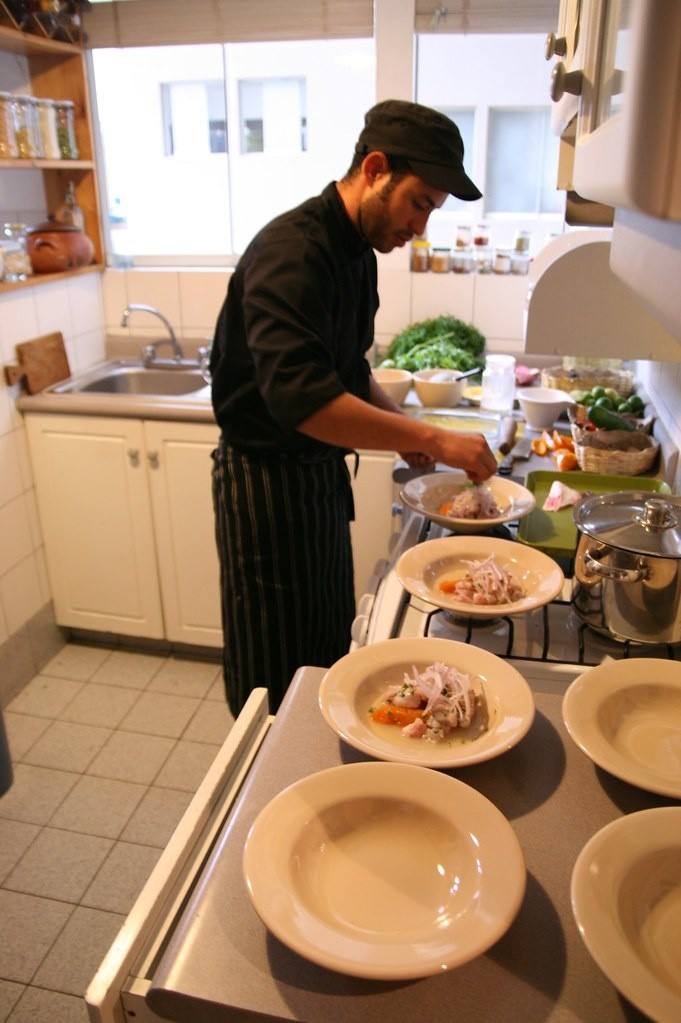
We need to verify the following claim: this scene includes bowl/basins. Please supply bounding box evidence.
[412,368,467,408]
[517,387,576,432]
[371,369,412,407]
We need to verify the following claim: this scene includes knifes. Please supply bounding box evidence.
[498,437,533,475]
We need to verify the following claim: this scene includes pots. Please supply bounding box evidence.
[25,214,95,274]
[570,491,680,647]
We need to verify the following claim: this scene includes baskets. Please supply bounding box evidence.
[567,401,637,421]
[542,365,635,416]
[572,431,660,474]
[571,415,655,439]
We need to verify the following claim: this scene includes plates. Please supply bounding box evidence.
[570,806,680,1023]
[242,761,527,981]
[463,386,481,406]
[318,637,537,770]
[395,535,565,621]
[399,471,537,532]
[561,657,681,800]
[516,470,673,561]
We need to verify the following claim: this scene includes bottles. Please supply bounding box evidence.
[480,354,516,421]
[0,222,33,283]
[60,180,85,233]
[0,90,79,159]
[411,222,531,277]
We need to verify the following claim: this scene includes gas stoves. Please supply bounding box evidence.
[388,480,680,679]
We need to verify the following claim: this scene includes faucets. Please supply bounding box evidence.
[120,304,182,360]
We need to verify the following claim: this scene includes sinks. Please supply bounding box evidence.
[80,367,210,398]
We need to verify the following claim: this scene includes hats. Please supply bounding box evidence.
[355,99,484,202]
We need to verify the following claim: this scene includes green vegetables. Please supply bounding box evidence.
[377,314,486,379]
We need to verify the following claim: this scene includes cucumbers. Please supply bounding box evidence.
[586,406,635,432]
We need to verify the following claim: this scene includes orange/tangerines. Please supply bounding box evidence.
[576,385,644,415]
[553,449,579,474]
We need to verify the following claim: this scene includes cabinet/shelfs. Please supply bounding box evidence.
[0,0,106,293]
[20,410,409,668]
[85,668,681,1023]
[545,0,681,228]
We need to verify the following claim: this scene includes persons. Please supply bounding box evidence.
[209,99,499,725]
